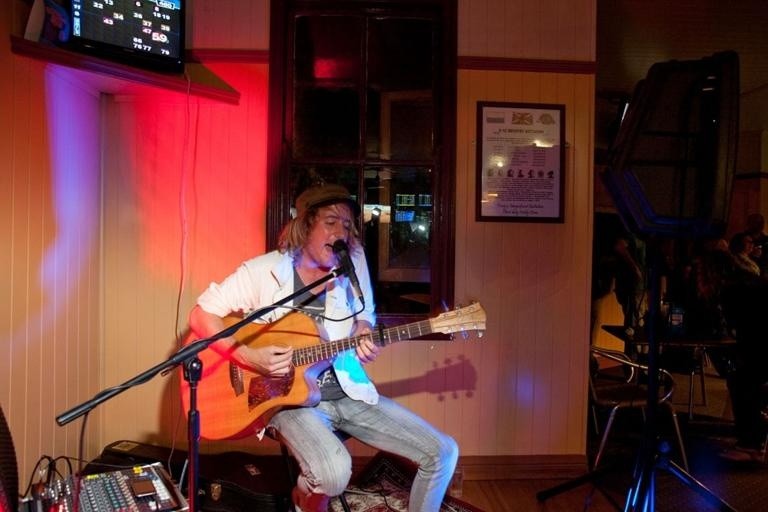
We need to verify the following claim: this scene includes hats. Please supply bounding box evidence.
[295,183,362,217]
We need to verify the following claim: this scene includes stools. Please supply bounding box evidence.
[667,333,707,421]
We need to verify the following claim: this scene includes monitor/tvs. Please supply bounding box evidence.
[67,0,189,75]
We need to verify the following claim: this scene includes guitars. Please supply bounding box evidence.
[178,300,486,441]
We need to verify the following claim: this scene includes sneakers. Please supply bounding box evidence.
[290,484,329,512]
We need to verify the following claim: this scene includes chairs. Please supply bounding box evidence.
[262,426,355,512]
[588,343,695,474]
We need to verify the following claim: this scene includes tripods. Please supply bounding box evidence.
[531,252,738,512]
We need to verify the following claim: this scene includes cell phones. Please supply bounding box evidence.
[128,474,157,498]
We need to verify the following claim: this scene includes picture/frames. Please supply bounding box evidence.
[475,101,565,223]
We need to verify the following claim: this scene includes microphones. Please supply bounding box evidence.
[330,238,366,303]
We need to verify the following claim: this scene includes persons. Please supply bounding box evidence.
[591,209,767,470]
[188,184,460,512]
